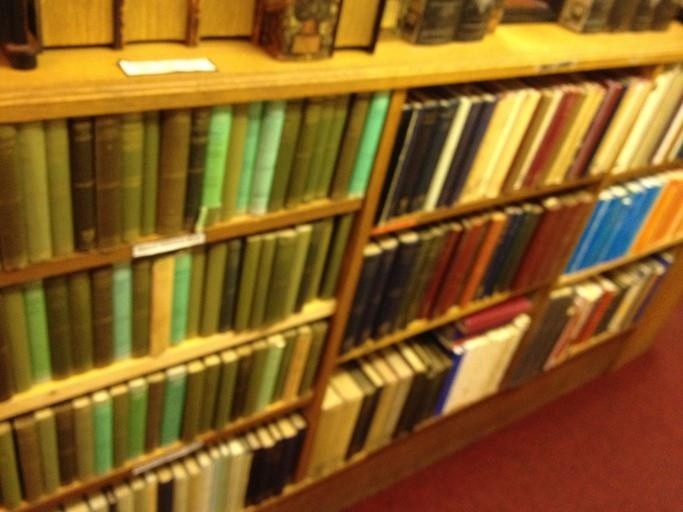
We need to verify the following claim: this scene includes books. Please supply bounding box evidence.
[2,1,682,512]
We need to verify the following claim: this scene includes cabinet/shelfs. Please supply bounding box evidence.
[1,19,683,511]
